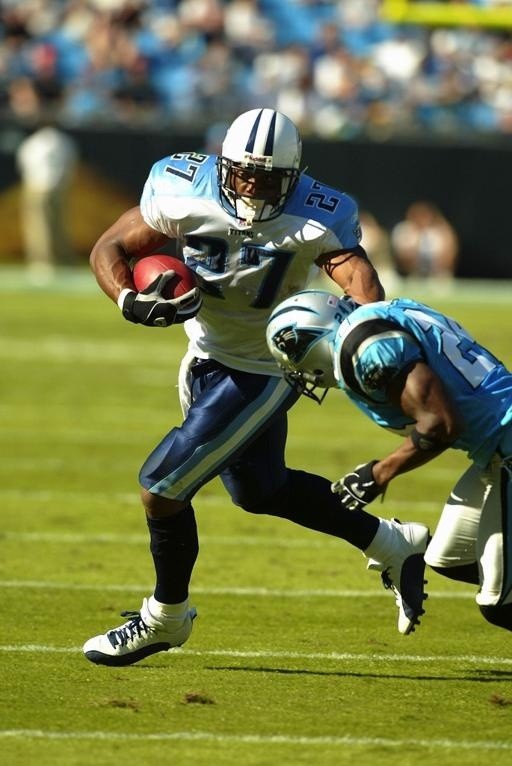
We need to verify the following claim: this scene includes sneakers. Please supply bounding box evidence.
[82,598,198,667]
[366,516,431,637]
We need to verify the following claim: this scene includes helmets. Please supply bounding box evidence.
[216,108,301,224]
[264,288,355,405]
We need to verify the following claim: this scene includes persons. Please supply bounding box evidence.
[79,104,437,668]
[1,1,512,288]
[265,286,511,632]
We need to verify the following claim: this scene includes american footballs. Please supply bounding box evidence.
[133,254,199,302]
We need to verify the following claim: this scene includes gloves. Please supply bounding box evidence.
[122,271,204,327]
[331,459,388,515]
